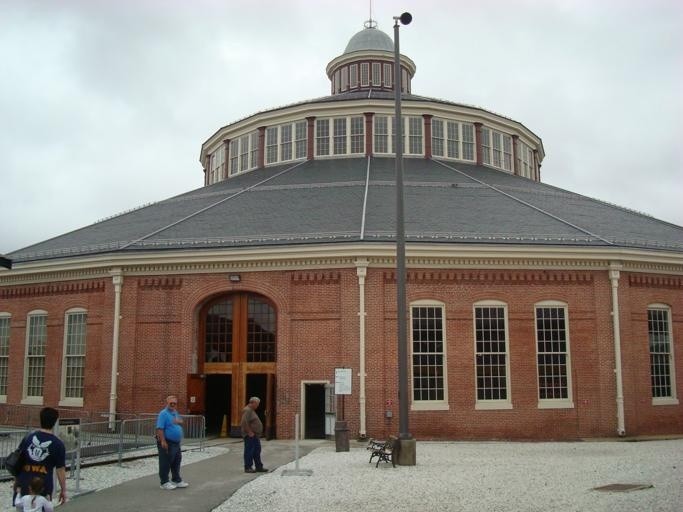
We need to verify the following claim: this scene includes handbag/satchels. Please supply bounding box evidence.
[5,448,22,476]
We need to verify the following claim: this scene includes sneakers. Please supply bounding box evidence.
[160,482,176,490]
[172,481,188,488]
[256,468,268,472]
[245,468,256,473]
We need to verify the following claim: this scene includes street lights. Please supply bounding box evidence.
[391,11,418,466]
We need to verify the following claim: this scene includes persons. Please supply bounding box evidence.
[15,476,53,512]
[241,397,268,472]
[12,407,67,508]
[155,396,189,490]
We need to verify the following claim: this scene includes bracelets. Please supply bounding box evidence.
[180,420,184,424]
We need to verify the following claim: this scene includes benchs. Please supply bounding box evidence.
[368,433,402,469]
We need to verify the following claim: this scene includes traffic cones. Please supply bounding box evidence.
[218,415,229,438]
